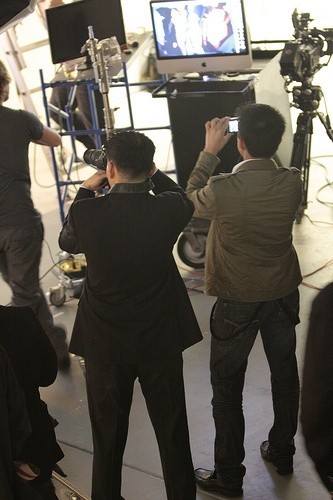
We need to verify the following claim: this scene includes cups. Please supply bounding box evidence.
[63,64,78,78]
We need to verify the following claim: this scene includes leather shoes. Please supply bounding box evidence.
[259,440,294,476]
[193,467,244,498]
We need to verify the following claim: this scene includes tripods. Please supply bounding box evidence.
[289,86,333,224]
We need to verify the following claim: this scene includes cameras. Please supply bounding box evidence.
[227,117,240,133]
[83,145,107,171]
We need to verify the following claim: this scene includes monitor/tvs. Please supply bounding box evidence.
[45,0,126,70]
[149,0,253,80]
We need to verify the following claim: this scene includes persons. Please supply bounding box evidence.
[301,282,332,499]
[0,62,71,374]
[185,104,302,499]
[58,130,203,500]
[1,305,66,499]
[39,0,111,149]
